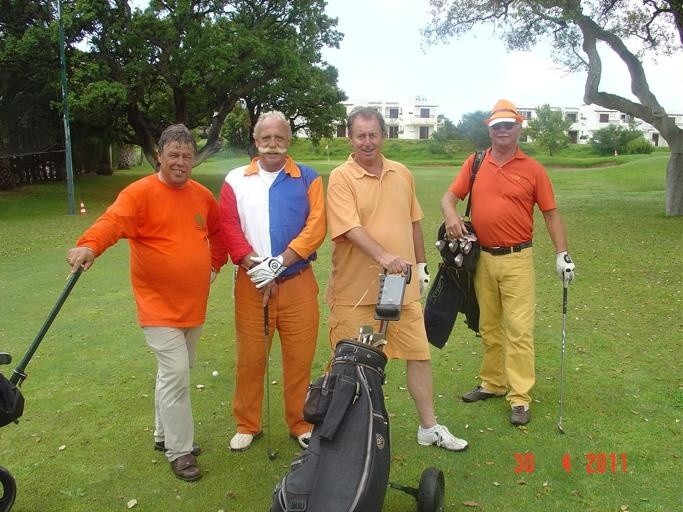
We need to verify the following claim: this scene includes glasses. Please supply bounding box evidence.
[493,124,516,130]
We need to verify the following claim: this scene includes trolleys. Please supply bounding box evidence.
[0,257,81,512]
[265,263,445,512]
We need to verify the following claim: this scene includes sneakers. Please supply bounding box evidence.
[418,425,468,451]
[462,386,494,402]
[511,406,530,425]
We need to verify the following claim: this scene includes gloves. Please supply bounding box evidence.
[417,263,429,296]
[556,250,575,288]
[247,255,288,289]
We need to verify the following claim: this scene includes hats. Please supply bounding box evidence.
[484,99,524,126]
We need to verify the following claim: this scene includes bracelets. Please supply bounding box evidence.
[248,261,260,270]
[87,247,94,254]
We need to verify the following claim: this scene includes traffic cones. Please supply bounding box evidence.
[77,200,87,217]
[614,150,618,156]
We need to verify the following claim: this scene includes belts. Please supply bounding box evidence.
[482,240,532,255]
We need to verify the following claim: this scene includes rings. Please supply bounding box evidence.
[67,257,72,261]
[449,231,453,236]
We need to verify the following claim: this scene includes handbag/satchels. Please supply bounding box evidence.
[435,218,480,278]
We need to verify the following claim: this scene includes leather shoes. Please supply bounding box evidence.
[230,430,263,452]
[297,431,313,449]
[171,453,202,481]
[155,440,201,457]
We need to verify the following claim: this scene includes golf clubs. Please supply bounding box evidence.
[264,299,280,459]
[435,232,478,268]
[557,274,568,435]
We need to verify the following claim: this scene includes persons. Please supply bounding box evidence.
[67,122,229,484]
[219,110,328,453]
[325,103,470,454]
[437,97,576,428]
